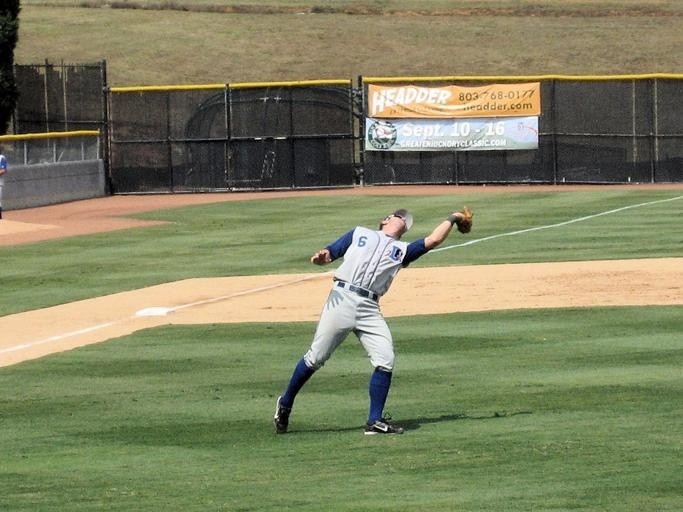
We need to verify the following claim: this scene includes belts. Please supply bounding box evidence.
[335,280,379,303]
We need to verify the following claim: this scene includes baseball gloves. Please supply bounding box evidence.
[452,205,473,233]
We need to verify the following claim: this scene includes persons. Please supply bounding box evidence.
[1,154,8,220]
[271,208,475,435]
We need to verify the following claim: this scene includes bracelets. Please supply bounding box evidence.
[446,214,458,226]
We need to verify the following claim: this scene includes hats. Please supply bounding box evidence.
[382,208,414,231]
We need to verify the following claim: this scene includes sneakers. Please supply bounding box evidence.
[273,395,291,433]
[364,419,404,435]
